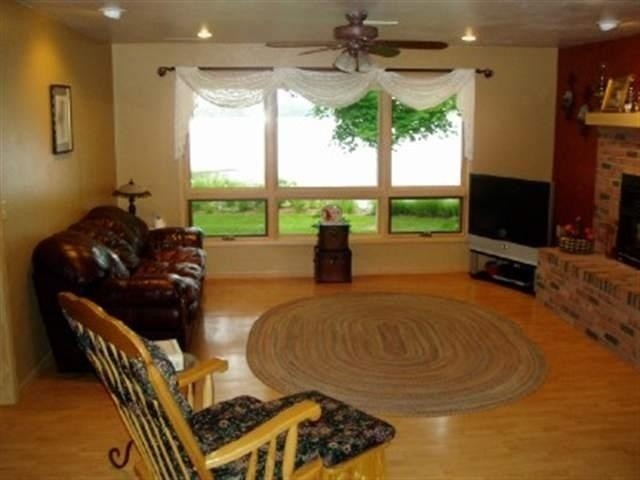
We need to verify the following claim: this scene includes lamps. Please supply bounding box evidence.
[595,5,620,32]
[99,0,126,19]
[332,45,374,72]
[461,25,476,41]
[114,179,152,213]
[197,23,212,38]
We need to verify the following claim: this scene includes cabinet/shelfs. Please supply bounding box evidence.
[469,249,538,296]
[314,225,353,283]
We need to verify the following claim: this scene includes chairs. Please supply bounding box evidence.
[58,289,325,480]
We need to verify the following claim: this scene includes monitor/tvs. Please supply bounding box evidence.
[467,171,556,249]
[613,172,640,269]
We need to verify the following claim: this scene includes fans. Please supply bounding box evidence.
[266,8,448,59]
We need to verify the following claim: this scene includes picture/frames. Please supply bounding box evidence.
[600,73,635,113]
[49,83,73,155]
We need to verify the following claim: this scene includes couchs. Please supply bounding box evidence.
[32,205,208,376]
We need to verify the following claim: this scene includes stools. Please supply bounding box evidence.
[262,389,395,480]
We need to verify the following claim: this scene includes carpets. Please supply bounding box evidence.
[247,291,546,418]
